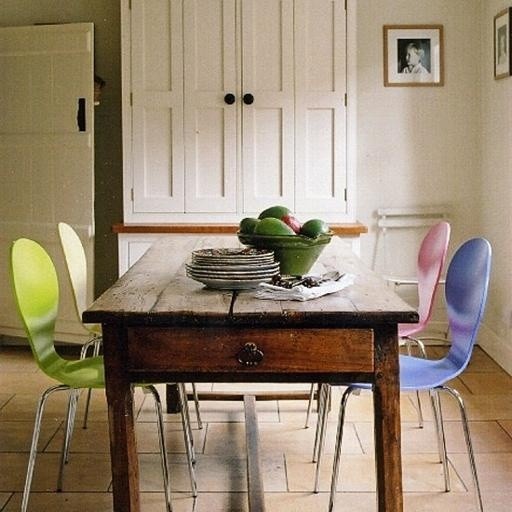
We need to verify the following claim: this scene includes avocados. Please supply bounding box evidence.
[239,205,328,239]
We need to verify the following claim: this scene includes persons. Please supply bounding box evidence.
[396,40,430,74]
[498,32,506,64]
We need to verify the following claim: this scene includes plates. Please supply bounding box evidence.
[184,247,282,291]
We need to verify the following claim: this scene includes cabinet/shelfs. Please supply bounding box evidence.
[119,0,356,225]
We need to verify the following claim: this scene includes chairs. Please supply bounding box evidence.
[10,235,200,510]
[313,237,493,511]
[58,222,203,467]
[306,219,452,464]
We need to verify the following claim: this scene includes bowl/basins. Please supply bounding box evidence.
[235,228,336,278]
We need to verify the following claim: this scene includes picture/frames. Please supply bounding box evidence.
[382,24,445,88]
[492,6,511,81]
